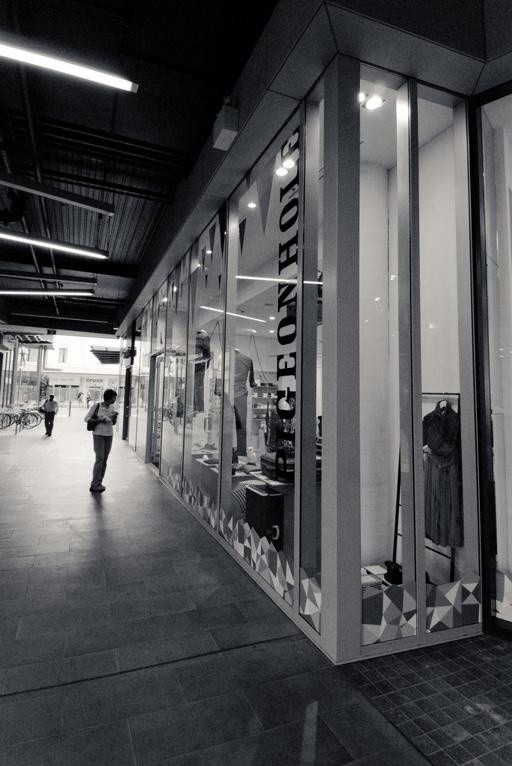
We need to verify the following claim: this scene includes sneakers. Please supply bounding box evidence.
[90,485,106,492]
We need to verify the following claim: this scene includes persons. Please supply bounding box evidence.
[42,394,59,437]
[235,349,259,461]
[77,391,92,410]
[83,389,119,492]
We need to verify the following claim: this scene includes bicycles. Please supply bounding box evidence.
[0,402,41,429]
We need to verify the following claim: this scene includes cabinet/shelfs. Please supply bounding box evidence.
[251,383,278,420]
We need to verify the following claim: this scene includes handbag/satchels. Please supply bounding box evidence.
[87,413,98,431]
[39,407,46,414]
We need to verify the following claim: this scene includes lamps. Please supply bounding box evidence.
[0,38,143,302]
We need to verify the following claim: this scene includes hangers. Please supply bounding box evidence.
[439,391,449,409]
